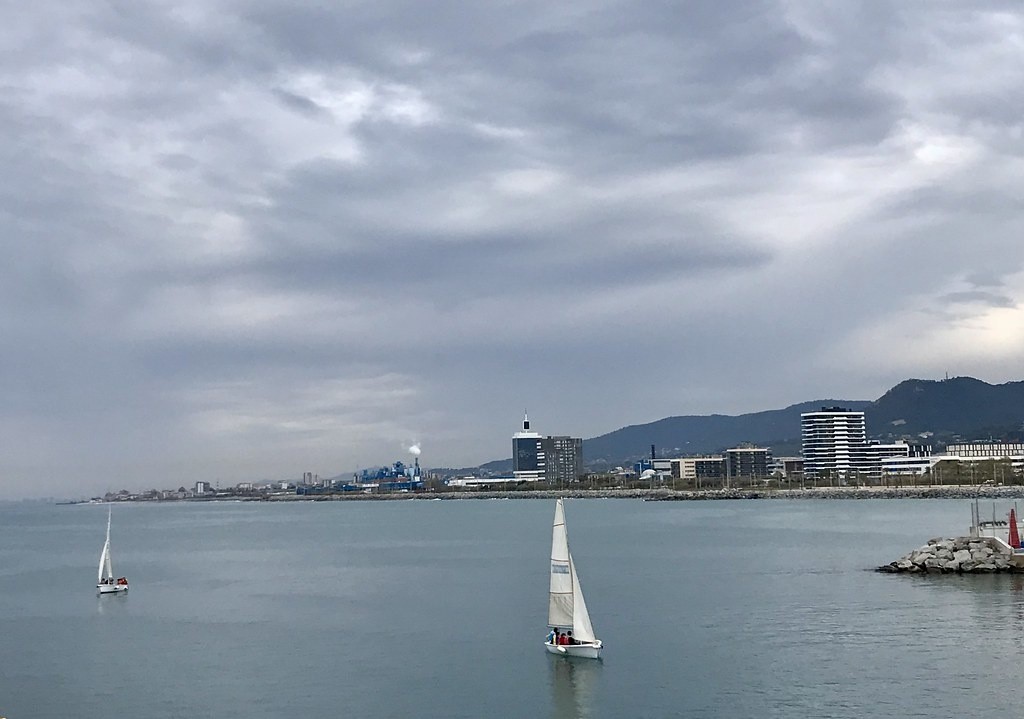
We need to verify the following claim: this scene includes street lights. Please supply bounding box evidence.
[975,479,994,538]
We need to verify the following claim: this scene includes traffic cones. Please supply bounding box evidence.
[1007,509,1021,550]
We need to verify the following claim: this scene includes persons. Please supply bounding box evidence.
[549,628,575,645]
[117,577,127,584]
[102,578,108,584]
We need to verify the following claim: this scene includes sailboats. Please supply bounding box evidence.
[94,508,129,594]
[544,497,603,660]
